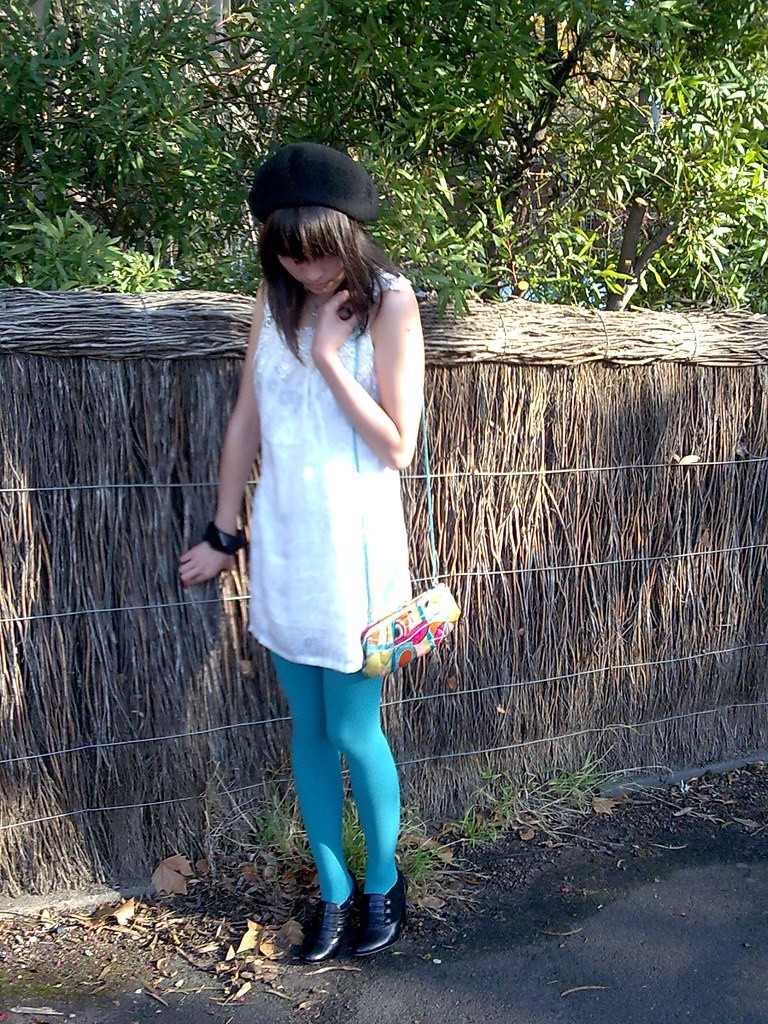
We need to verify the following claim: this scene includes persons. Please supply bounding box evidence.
[180,141,427,961]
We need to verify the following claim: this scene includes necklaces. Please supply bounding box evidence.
[309,293,322,319]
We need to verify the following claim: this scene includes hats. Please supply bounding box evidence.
[248,142,380,221]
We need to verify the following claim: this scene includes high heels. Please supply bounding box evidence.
[296,869,361,964]
[350,867,409,958]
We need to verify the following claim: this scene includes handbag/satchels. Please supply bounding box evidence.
[361,583,461,678]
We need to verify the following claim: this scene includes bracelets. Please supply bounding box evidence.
[202,522,246,553]
